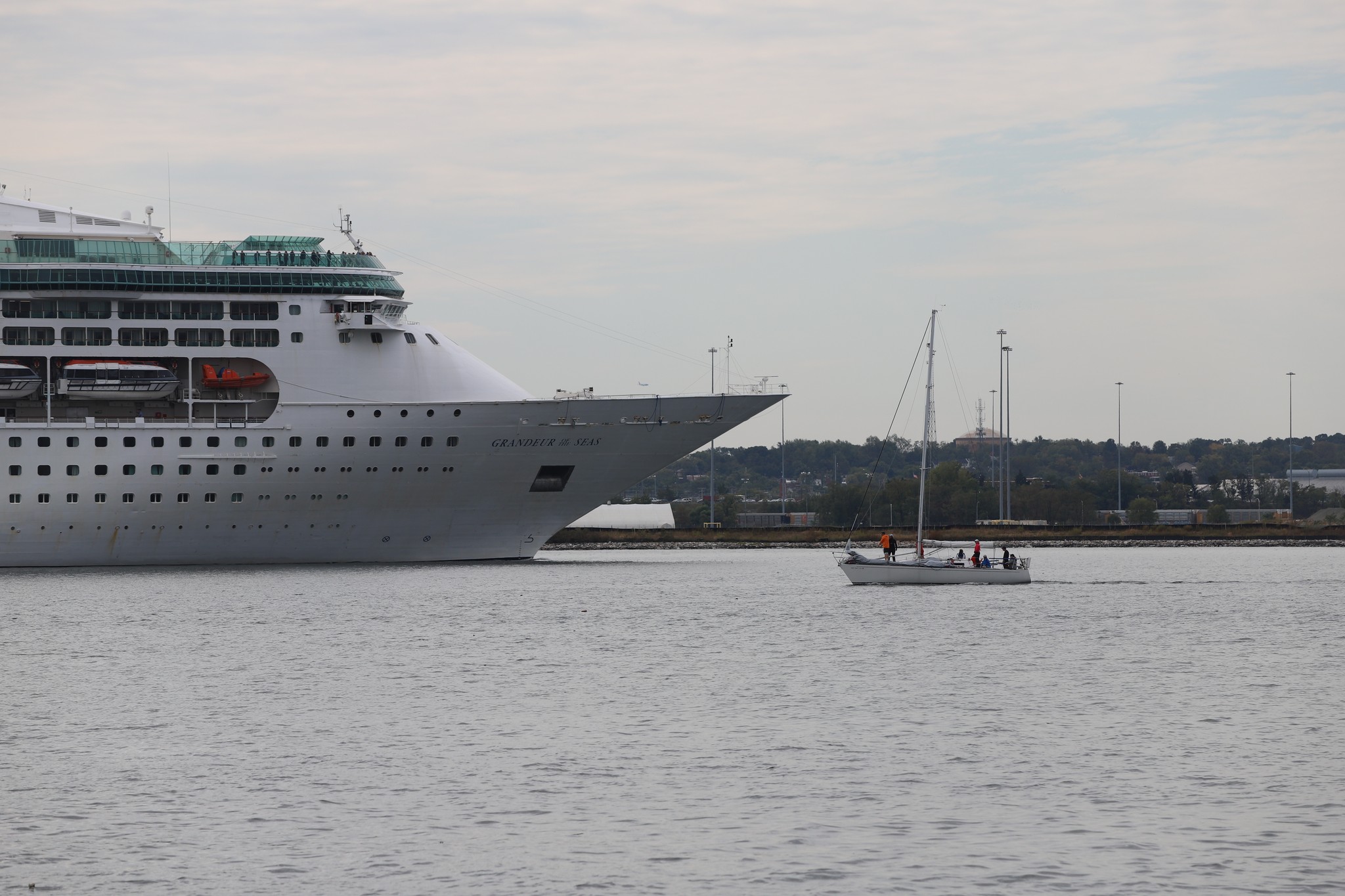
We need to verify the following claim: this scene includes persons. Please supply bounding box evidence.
[916,536,924,558]
[231,250,373,266]
[1001,546,1017,570]
[956,549,966,559]
[878,532,897,562]
[971,539,991,568]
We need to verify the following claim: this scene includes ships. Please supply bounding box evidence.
[0,181,794,569]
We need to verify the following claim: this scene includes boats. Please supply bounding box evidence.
[0,363,42,400]
[54,359,181,401]
[201,362,270,389]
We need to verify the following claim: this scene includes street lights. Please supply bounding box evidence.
[708,347,718,528]
[1286,372,1296,517]
[778,385,787,514]
[1115,382,1124,510]
[989,329,1013,520]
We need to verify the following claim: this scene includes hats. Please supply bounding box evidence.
[1010,554,1014,557]
[881,532,885,535]
[974,539,979,542]
[890,534,893,536]
[983,555,987,557]
[1001,546,1006,549]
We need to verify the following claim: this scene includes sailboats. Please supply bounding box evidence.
[831,308,1034,585]
